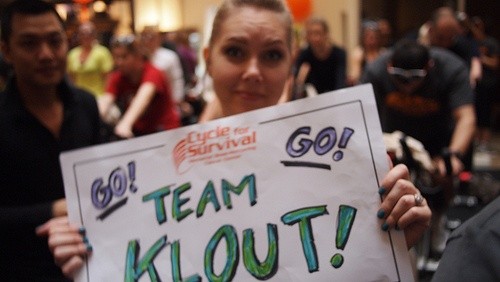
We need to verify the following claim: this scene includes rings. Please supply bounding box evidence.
[414,193,424,206]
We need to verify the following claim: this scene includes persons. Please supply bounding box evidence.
[0,0,102,282]
[64,22,217,142]
[282,6,500,282]
[35,0,432,282]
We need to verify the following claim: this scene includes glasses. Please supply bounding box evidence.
[111,33,135,44]
